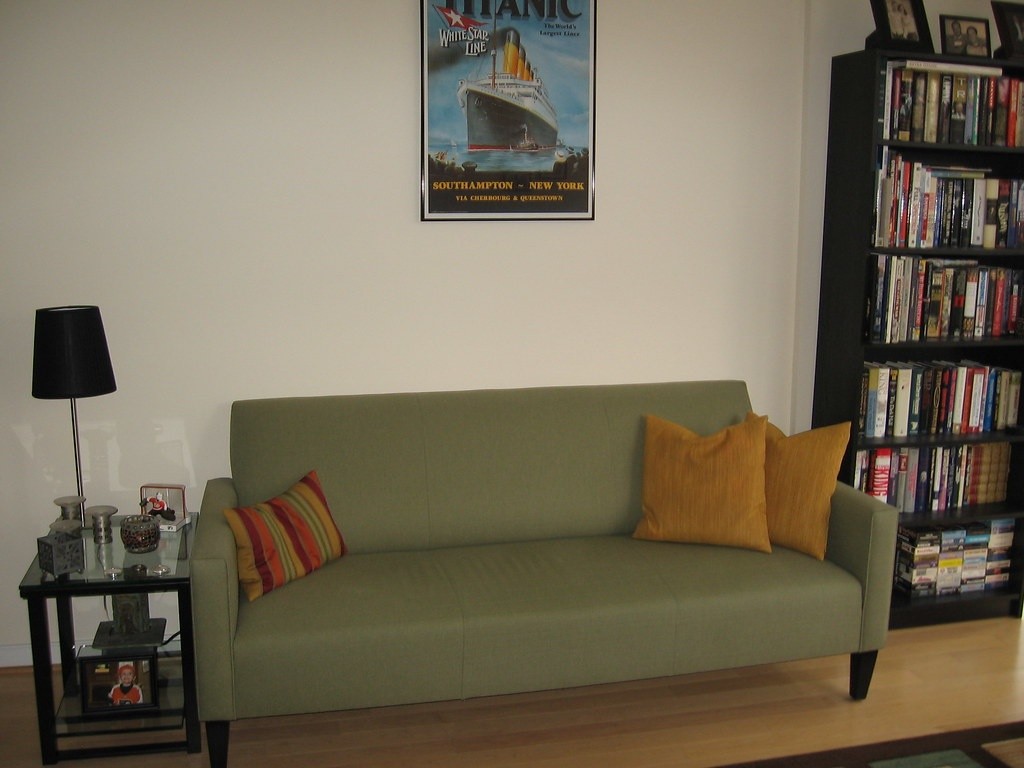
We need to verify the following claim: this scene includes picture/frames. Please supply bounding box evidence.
[869,0,935,53]
[990,1,1024,47]
[939,14,992,59]
[420,0,598,220]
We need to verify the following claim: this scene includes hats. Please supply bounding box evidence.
[119,665,135,675]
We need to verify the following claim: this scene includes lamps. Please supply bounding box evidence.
[31,305,116,528]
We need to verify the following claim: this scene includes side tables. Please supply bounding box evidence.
[17,512,202,766]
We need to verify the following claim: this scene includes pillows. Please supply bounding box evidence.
[633,412,772,554]
[222,469,350,601]
[744,410,851,563]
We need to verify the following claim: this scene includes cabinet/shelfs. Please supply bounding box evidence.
[811,49,1024,631]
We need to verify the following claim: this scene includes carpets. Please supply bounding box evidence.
[714,718,1024,768]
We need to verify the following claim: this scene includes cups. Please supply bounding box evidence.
[120,513,160,553]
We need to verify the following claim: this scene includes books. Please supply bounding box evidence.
[854,67,1024,598]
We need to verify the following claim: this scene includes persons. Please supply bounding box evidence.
[887,0,917,41]
[946,21,987,58]
[107,664,143,706]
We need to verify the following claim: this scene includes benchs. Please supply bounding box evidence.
[187,381,900,768]
